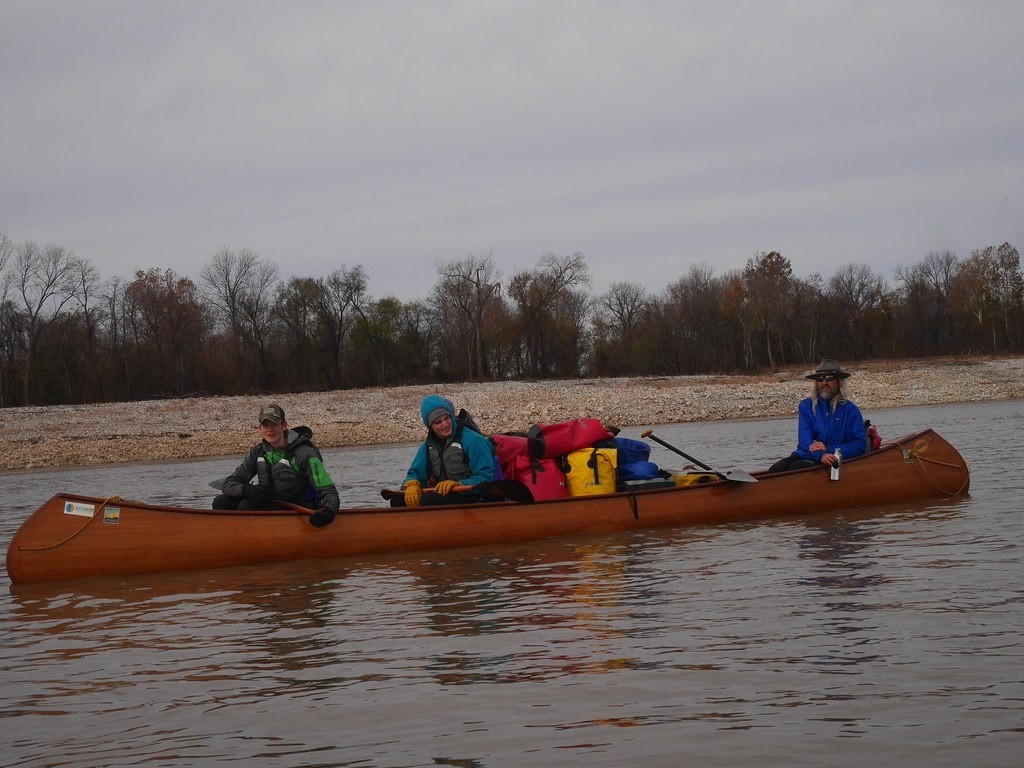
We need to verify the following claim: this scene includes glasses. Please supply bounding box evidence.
[815,375,836,382]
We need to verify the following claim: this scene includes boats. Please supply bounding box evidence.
[6,426,971,586]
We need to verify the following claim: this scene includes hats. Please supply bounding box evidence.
[805,358,852,379]
[258,404,284,425]
[426,407,451,426]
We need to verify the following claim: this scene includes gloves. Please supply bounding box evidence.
[404,479,422,507]
[244,485,266,498]
[434,480,462,495]
[309,508,334,528]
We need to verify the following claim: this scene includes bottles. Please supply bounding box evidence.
[830,447,842,481]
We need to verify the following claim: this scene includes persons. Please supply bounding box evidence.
[213,402,340,527]
[767,358,868,475]
[390,394,511,508]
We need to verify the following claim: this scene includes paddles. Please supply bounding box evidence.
[379,479,536,505]
[639,429,760,483]
[207,477,319,517]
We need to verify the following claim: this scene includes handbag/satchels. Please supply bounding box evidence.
[487,416,672,502]
